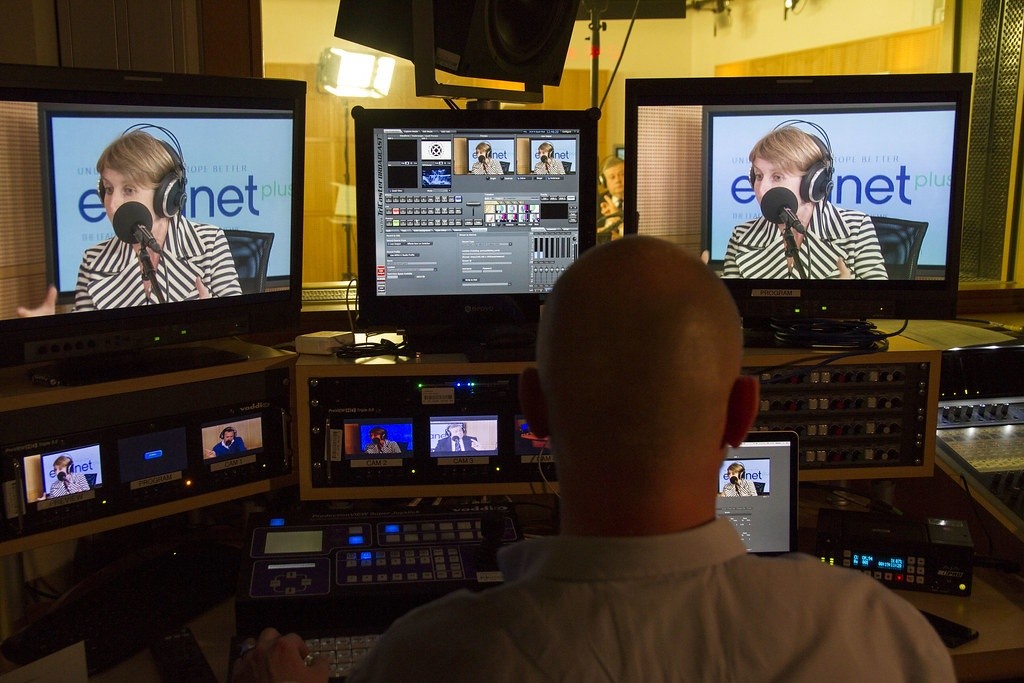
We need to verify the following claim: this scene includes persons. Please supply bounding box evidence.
[434,424,478,452]
[205,427,247,459]
[533,142,567,174]
[229,234,958,683]
[722,463,758,497]
[719,126,890,279]
[36,455,92,501]
[596,156,624,244]
[471,142,504,174]
[16,130,243,317]
[363,427,402,453]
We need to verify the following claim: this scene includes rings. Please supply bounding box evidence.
[238,644,256,656]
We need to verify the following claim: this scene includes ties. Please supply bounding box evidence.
[455,439,461,452]
[617,199,623,208]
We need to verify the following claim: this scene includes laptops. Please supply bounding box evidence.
[711,432,800,557]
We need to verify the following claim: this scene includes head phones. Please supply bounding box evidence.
[219,427,237,439]
[380,427,387,440]
[600,155,618,189]
[445,425,465,437]
[737,462,745,479]
[545,141,555,158]
[65,457,74,475]
[750,119,834,203]
[485,141,492,158]
[121,122,188,218]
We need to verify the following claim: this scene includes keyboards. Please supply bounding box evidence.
[300,633,386,679]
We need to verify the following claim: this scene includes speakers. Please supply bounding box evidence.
[334,0,580,86]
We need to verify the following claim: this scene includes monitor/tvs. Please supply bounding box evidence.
[0,354,300,557]
[621,72,973,330]
[294,354,562,502]
[354,109,597,355]
[0,63,305,386]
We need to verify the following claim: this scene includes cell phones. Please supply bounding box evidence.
[918,609,980,649]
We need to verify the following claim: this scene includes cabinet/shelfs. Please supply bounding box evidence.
[0,325,1024,560]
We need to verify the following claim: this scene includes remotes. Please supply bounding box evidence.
[149,627,219,683]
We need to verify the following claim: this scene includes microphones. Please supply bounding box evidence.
[541,155,550,166]
[478,155,487,165]
[730,475,740,487]
[452,436,460,443]
[57,471,73,484]
[372,437,381,451]
[112,201,162,255]
[226,440,232,448]
[760,187,805,234]
